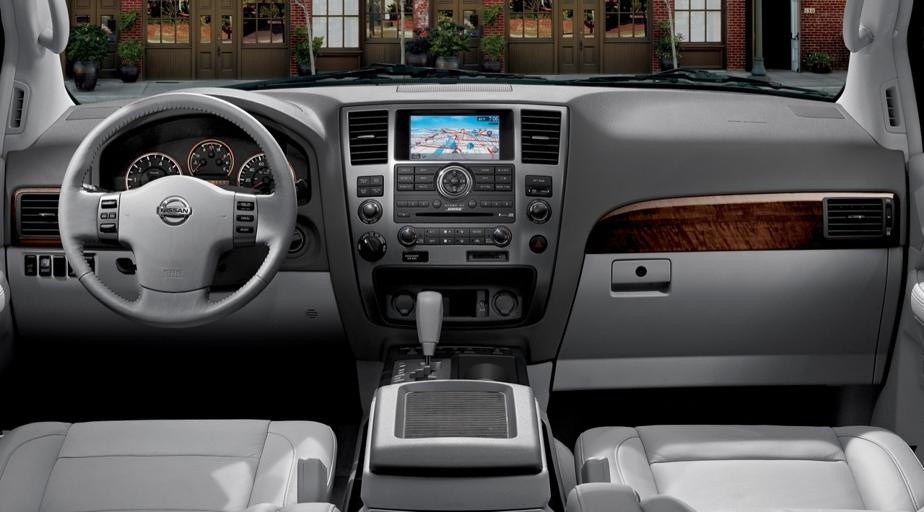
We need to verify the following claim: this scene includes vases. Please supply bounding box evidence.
[293,26,324,78]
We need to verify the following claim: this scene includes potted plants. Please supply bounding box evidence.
[65,19,147,92]
[653,16,682,75]
[806,51,834,74]
[406,6,504,73]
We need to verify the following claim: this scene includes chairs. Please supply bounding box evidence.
[1,418,342,509]
[563,418,922,508]
